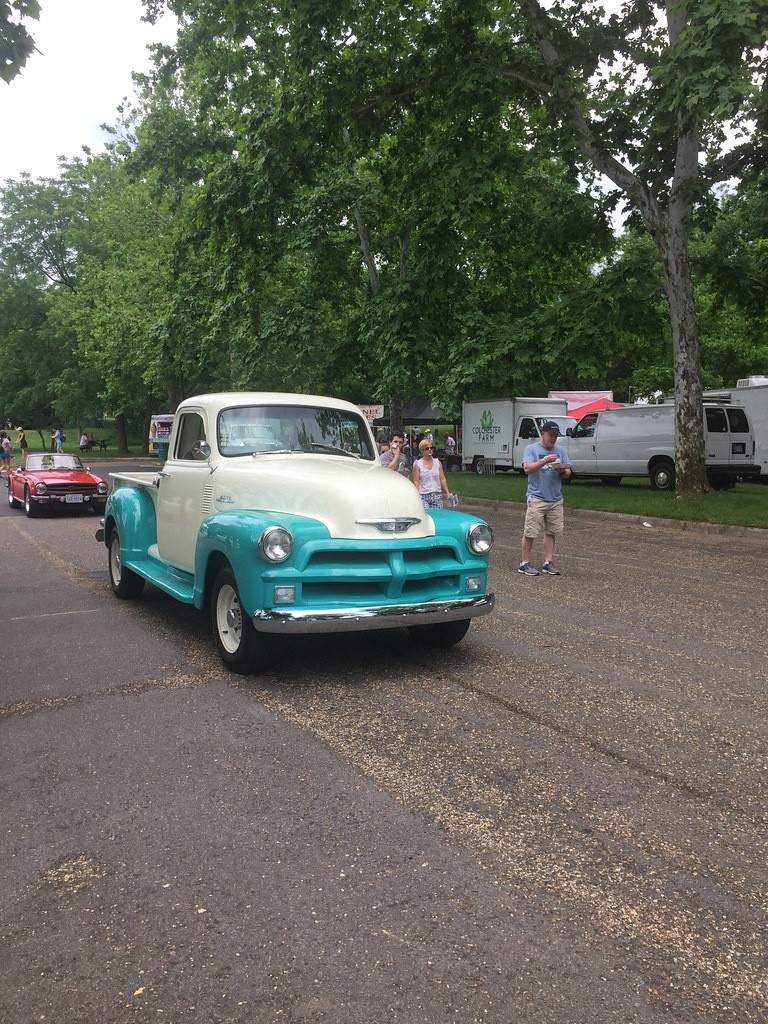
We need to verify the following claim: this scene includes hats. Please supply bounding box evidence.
[542,421,560,433]
[424,429,431,432]
[16,426,22,430]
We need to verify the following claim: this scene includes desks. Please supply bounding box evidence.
[97,439,110,451]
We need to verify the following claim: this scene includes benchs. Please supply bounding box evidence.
[80,447,92,453]
[104,443,111,446]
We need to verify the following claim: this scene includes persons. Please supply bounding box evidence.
[52,427,64,453]
[0,431,15,478]
[16,427,29,459]
[586,421,595,437]
[518,421,572,575]
[371,418,456,509]
[80,433,94,452]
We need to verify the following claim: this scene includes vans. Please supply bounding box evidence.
[555,376,768,491]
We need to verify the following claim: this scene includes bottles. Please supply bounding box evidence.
[447,493,453,507]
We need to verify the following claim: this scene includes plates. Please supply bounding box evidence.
[548,462,572,468]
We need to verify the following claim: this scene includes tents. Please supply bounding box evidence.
[568,396,626,420]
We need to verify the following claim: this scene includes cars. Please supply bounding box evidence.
[5,453,109,518]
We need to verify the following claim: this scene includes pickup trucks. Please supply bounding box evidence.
[96,392,495,675]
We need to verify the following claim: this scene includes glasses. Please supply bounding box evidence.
[425,447,434,450]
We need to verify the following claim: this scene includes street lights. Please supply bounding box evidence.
[629,386,637,403]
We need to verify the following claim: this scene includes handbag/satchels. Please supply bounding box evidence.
[57,434,66,442]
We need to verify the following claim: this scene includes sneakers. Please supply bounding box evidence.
[0,473,4,477]
[518,561,539,576]
[542,561,560,575]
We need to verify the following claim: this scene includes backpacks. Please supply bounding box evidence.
[0,439,7,452]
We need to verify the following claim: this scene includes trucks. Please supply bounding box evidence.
[462,397,583,475]
